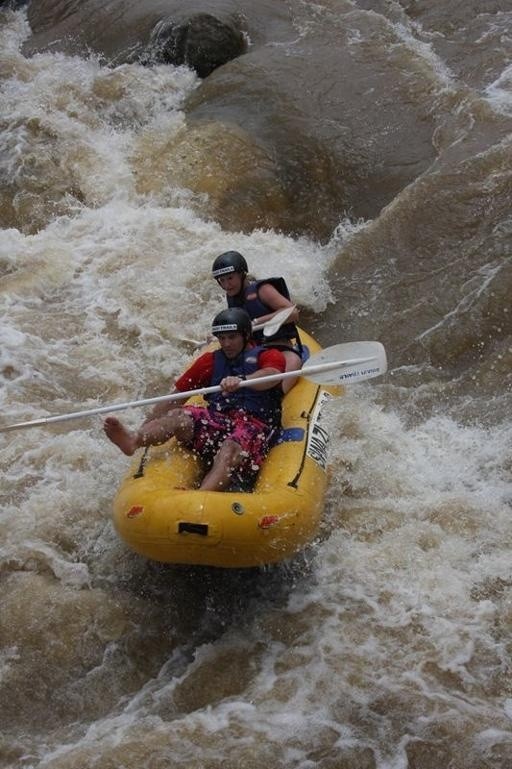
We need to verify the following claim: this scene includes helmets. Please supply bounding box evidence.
[212,307,251,341]
[212,251,248,279]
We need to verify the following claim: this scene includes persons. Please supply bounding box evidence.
[211,250,304,393]
[103,308,286,490]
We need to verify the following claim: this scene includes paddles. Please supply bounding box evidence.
[177,303,296,356]
[0,340,388,433]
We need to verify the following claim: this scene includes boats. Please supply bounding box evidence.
[112,324,345,568]
